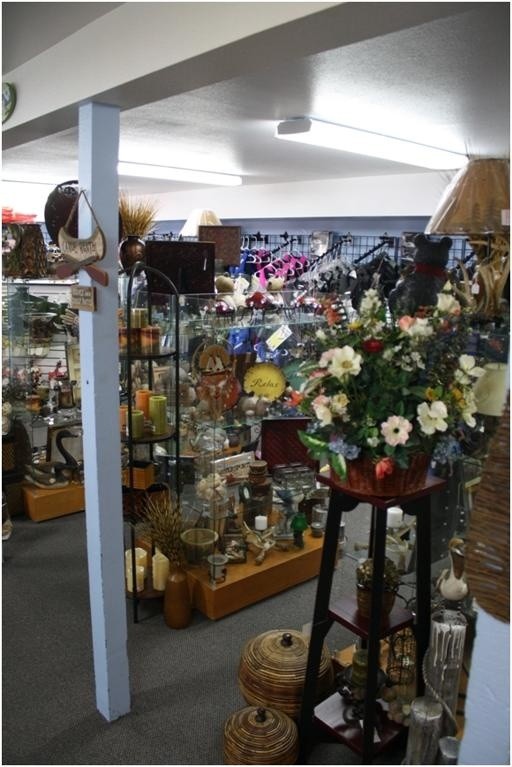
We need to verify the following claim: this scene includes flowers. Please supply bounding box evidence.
[280,289,483,484]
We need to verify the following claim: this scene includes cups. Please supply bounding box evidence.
[147,395,168,436]
[135,389,152,433]
[118,404,134,434]
[125,410,144,439]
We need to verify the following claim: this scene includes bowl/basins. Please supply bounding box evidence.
[180,528,220,564]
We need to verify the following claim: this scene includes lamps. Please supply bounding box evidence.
[271,116,468,171]
[422,155,509,310]
[465,361,509,420]
[116,158,247,188]
[179,209,224,237]
